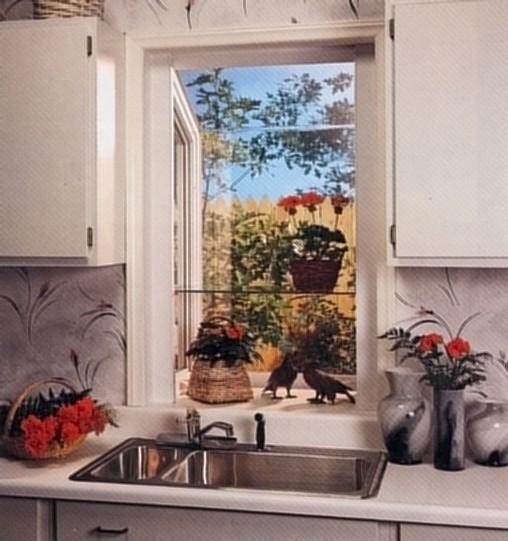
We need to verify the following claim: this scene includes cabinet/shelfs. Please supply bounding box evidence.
[2,18,126,273]
[384,2,507,270]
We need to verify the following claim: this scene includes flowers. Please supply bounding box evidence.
[187,317,252,365]
[20,390,118,461]
[276,191,353,263]
[384,313,488,387]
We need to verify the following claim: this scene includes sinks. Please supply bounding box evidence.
[160,444,374,498]
[80,438,194,484]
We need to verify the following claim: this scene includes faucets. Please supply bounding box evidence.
[175,403,236,442]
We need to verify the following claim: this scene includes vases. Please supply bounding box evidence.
[289,255,341,292]
[374,367,506,470]
[182,357,253,403]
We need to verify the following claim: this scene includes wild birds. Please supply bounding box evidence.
[300,359,356,406]
[262,351,300,401]
